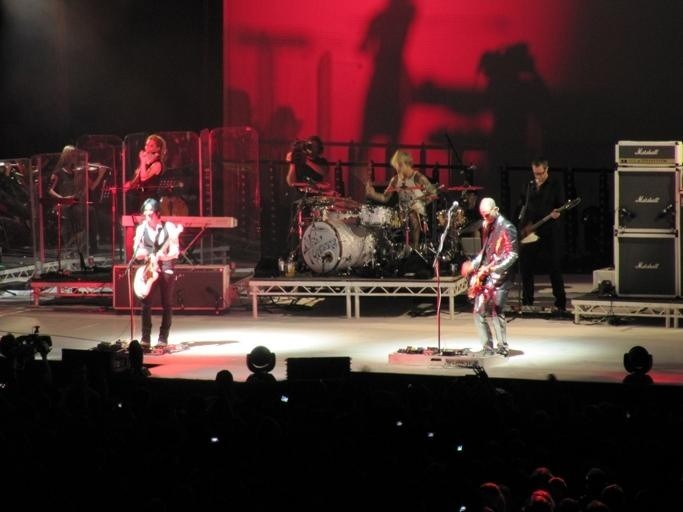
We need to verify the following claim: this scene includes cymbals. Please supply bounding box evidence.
[299,189,341,196]
[290,182,330,188]
[449,186,483,191]
[293,196,328,206]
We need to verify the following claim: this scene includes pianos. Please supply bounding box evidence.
[120,215,238,229]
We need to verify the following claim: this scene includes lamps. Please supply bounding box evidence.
[246,345,277,382]
[623,345,654,384]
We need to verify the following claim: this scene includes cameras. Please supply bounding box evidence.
[16,334,53,354]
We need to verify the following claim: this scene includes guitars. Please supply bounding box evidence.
[468,253,508,298]
[134,224,184,299]
[518,197,582,243]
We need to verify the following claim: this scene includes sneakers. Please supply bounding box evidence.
[497,347,511,356]
[481,348,494,356]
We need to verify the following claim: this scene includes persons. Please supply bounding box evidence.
[473,465,624,512]
[460,196,519,358]
[130,199,179,351]
[0,162,31,225]
[123,134,166,210]
[517,156,570,312]
[47,145,107,258]
[285,134,330,198]
[364,149,437,247]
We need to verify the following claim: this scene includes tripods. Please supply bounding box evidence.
[38,206,83,281]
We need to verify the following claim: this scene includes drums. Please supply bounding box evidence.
[439,208,466,228]
[361,205,397,227]
[311,197,363,221]
[301,221,377,272]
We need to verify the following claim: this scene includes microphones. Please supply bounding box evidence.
[448,201,459,213]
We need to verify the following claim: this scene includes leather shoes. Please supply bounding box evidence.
[157,340,167,348]
[140,341,151,349]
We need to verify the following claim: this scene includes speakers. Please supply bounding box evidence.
[112,265,231,313]
[204,286,223,300]
[285,356,351,381]
[60,347,131,373]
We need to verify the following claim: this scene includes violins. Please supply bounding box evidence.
[73,159,113,172]
[138,149,159,165]
